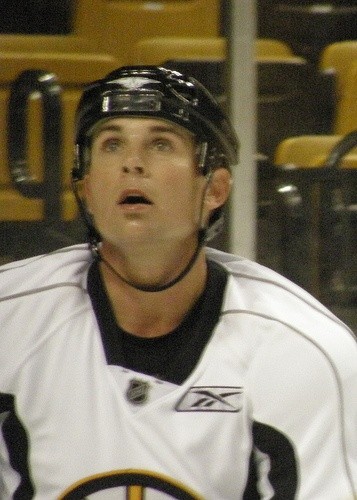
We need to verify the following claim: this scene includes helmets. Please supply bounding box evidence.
[74,64,240,181]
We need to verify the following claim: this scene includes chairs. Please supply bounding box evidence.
[0,0,357,294]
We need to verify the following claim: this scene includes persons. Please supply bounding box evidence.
[0,63,357,500]
